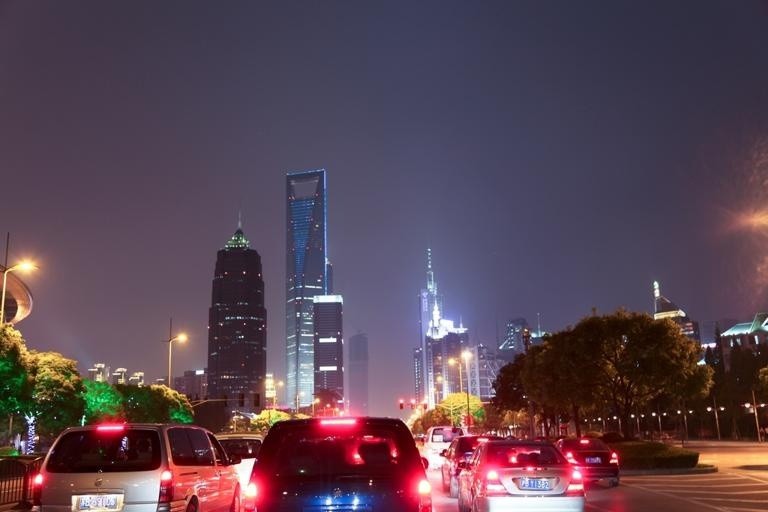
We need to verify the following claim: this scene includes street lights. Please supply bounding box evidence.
[583,390,766,442]
[273,381,345,417]
[0,261,41,326]
[167,332,189,388]
[407,350,474,433]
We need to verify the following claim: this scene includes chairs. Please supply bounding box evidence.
[358,442,393,467]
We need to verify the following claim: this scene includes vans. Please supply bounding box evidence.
[32,421,264,512]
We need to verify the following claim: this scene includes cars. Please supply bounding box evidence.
[554,436,621,487]
[414,426,588,512]
[0,456,42,506]
[243,417,433,512]
